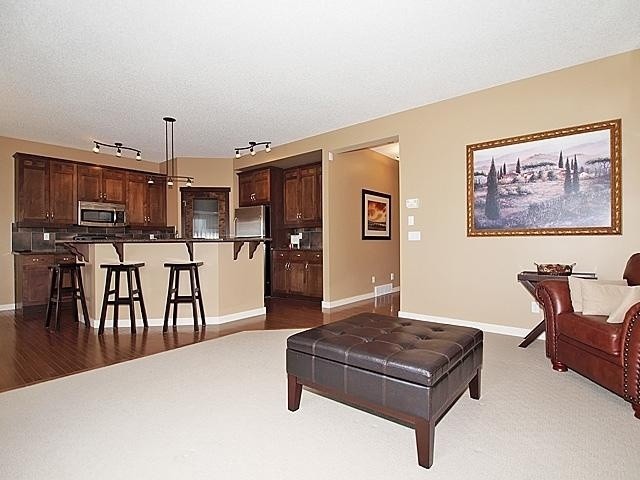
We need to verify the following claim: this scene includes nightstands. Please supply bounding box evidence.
[307,252,322,297]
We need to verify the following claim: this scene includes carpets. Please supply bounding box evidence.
[0,326,640,480]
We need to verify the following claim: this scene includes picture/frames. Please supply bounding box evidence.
[361,188,392,241]
[466,119,623,236]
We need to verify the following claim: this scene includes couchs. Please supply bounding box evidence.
[535,252,640,419]
[287,312,483,469]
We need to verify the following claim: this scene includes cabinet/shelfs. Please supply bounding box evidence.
[272,250,305,295]
[283,166,315,228]
[127,172,165,227]
[77,163,126,202]
[14,153,77,228]
[314,164,321,229]
[14,253,55,308]
[237,169,269,204]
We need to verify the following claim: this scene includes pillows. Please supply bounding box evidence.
[606,287,640,323]
[568,275,629,313]
[581,279,640,316]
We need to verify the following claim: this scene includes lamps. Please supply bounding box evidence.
[235,142,271,159]
[93,140,142,161]
[143,117,194,188]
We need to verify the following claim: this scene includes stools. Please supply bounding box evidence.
[163,263,204,332]
[45,263,90,329]
[98,264,148,336]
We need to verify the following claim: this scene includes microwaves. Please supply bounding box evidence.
[77,200,126,228]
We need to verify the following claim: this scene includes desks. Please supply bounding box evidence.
[518,272,598,349]
